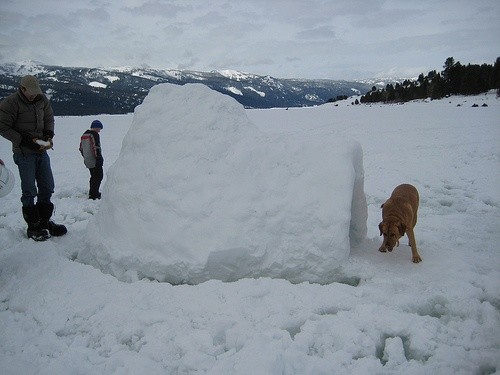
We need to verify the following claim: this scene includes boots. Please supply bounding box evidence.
[36,202,67,236]
[22,205,51,240]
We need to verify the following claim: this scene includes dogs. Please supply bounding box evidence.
[378,183,422,263]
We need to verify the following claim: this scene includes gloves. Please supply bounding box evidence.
[21,138,40,151]
[43,131,54,141]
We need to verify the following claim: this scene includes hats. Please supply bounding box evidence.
[21,75,43,95]
[91,120,103,129]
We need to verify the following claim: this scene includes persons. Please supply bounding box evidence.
[0,75,68,240]
[79,120,104,200]
